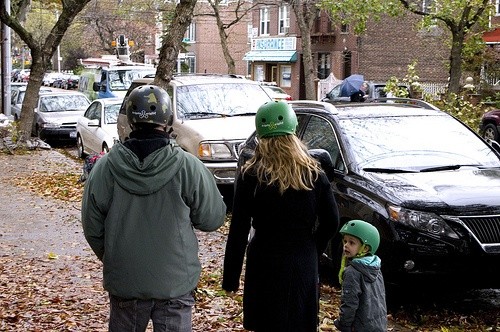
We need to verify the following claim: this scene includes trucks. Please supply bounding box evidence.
[79,64,156,97]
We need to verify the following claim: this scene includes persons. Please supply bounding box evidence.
[350,82,370,102]
[222,100,340,332]
[80,84,227,332]
[333,219,388,332]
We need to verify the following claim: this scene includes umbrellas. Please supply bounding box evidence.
[339,74,364,97]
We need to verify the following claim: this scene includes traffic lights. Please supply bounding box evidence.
[120,35,124,44]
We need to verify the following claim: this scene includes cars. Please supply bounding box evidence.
[10,88,52,121]
[77,97,125,158]
[262,85,292,101]
[479,110,499,148]
[11,69,79,90]
[32,92,92,145]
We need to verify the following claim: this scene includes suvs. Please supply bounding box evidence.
[321,82,409,103]
[116,72,274,208]
[237,98,500,311]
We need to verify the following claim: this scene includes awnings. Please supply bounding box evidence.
[242,51,297,62]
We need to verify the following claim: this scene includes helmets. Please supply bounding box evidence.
[127,85,174,130]
[340,219,381,255]
[254,101,297,136]
